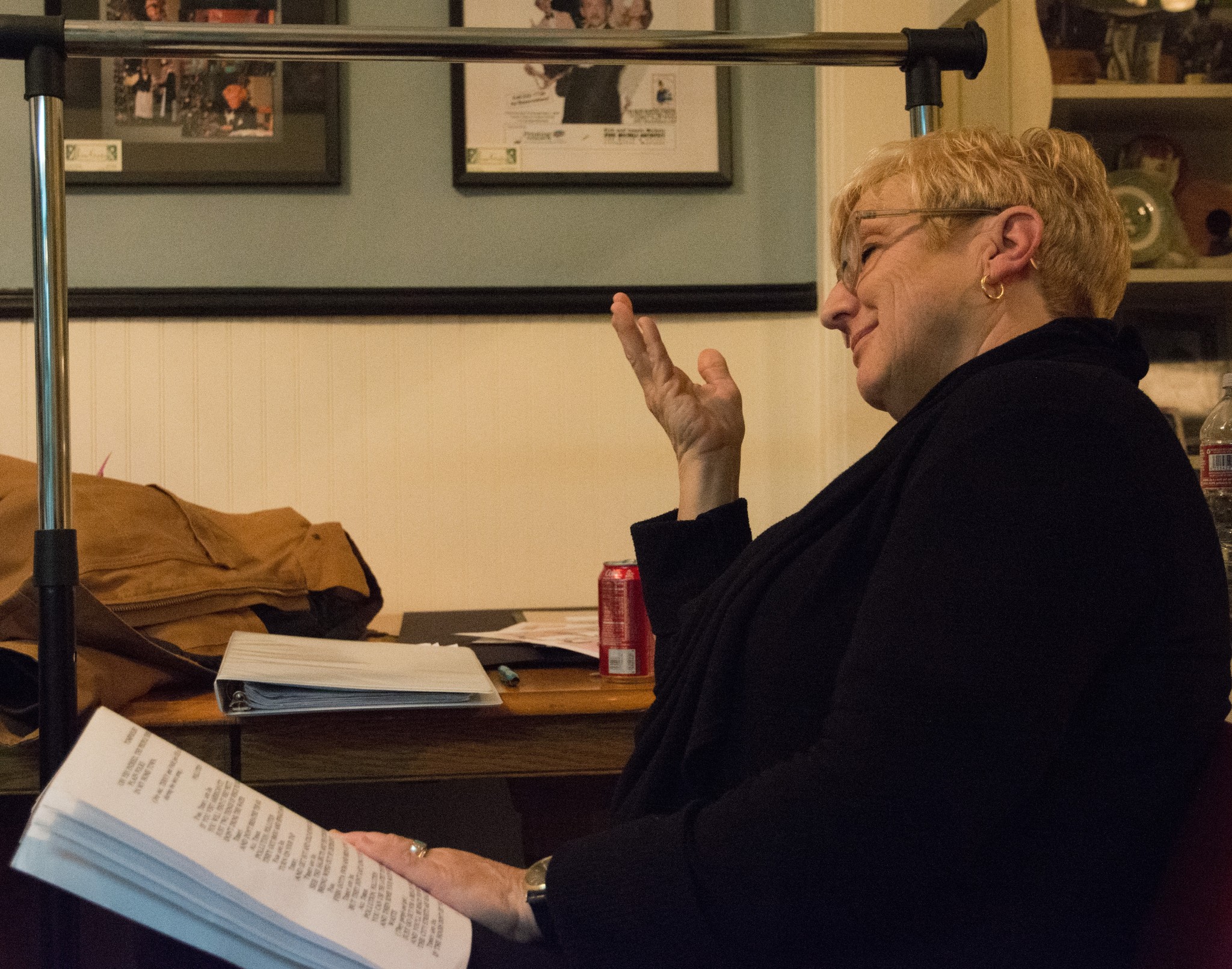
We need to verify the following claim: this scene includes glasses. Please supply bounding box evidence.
[841,203,1001,289]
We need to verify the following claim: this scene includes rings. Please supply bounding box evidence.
[410,840,427,856]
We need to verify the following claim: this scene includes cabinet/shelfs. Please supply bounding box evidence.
[928,0,1231,296]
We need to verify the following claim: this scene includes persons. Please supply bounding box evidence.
[135,1,259,131]
[326,125,1232,969]
[527,0,653,122]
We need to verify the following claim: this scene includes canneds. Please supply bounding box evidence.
[597,558,654,680]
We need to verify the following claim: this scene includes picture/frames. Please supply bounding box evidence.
[448,0,740,192]
[40,0,347,184]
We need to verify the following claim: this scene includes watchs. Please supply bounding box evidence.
[523,855,556,937]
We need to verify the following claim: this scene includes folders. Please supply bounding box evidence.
[213,630,504,717]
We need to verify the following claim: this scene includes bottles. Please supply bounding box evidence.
[1194,371,1232,624]
[1096,168,1176,266]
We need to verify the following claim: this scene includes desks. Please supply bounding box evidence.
[0,592,674,799]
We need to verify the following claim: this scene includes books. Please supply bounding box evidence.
[214,626,501,708]
[9,708,472,969]
